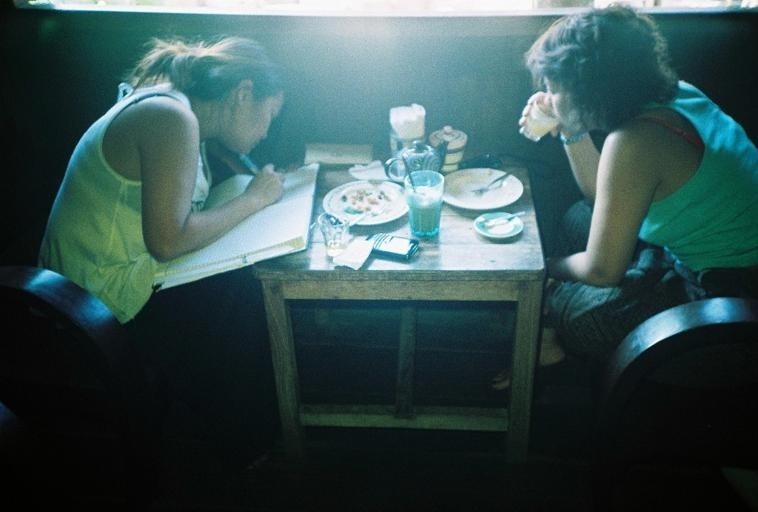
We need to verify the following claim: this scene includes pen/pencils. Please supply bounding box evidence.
[239,153,260,176]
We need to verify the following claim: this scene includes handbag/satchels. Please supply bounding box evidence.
[551,248,681,346]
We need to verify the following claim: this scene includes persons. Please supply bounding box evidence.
[39,30,293,479]
[489,5,758,414]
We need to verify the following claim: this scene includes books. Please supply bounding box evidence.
[155,163,318,292]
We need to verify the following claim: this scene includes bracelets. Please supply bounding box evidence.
[559,130,592,144]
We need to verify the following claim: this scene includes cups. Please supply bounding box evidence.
[318,210,351,256]
[520,101,559,142]
[389,130,424,155]
[427,126,469,170]
[404,170,444,238]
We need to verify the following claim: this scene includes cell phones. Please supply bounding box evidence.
[366,233,420,260]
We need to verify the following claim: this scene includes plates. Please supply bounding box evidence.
[322,180,410,226]
[442,167,524,210]
[473,212,524,239]
